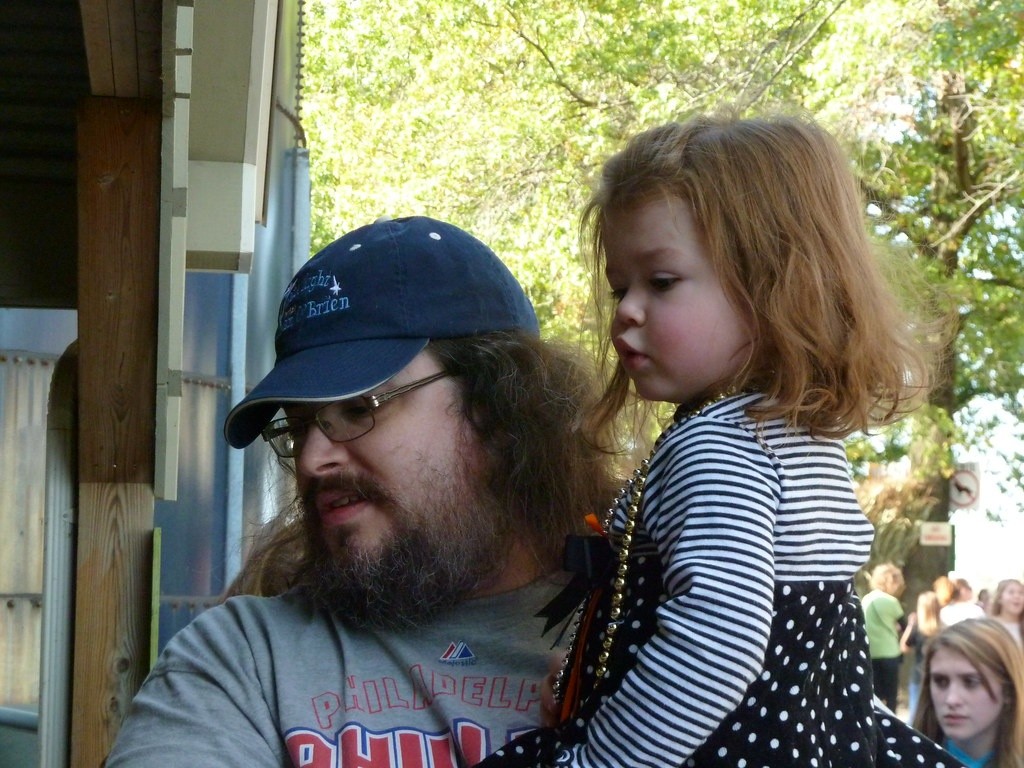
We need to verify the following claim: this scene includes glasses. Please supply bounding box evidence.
[262,363,471,457]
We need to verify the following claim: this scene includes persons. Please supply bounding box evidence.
[913,621,1023,767]
[858,565,1023,707]
[109,215,618,765]
[484,117,972,768]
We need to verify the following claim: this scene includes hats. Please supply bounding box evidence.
[223,216,542,450]
